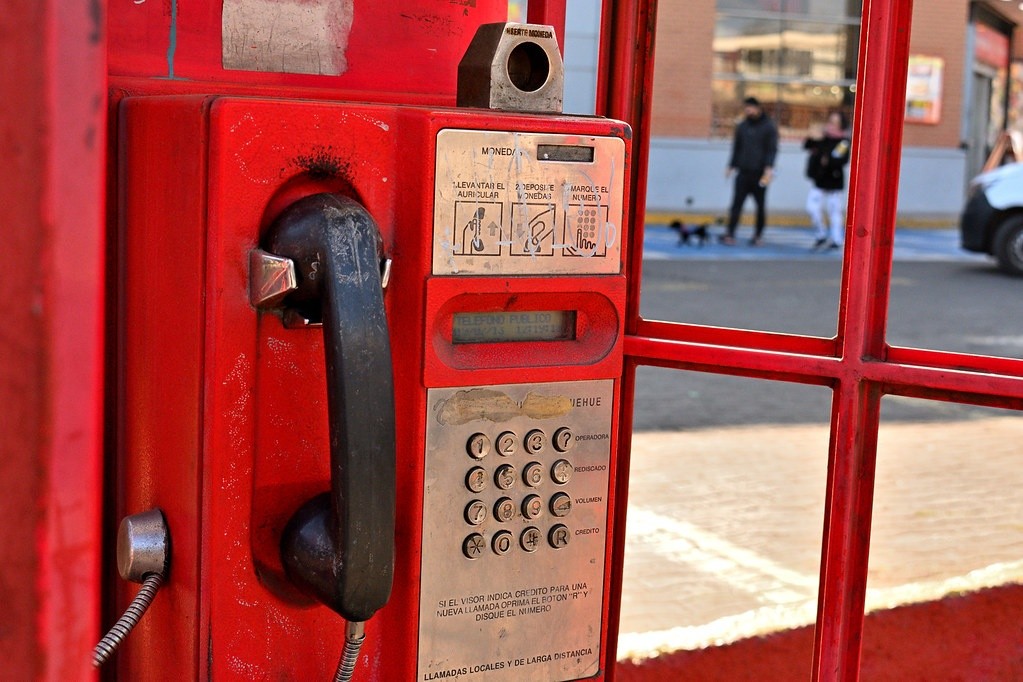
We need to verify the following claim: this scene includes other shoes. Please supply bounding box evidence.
[716,235,735,246]
[813,238,826,246]
[748,235,763,248]
[829,241,841,248]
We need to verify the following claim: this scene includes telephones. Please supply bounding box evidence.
[113,96,632,682]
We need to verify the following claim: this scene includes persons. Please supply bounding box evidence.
[717,97,778,248]
[802,111,850,250]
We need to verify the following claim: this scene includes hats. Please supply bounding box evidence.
[744,97,760,107]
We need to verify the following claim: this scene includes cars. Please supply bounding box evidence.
[959,161,1023,276]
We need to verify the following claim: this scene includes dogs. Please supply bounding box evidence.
[668,217,725,250]
